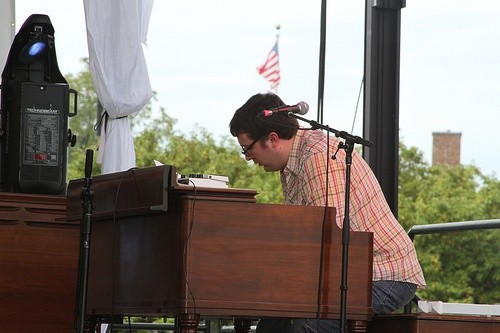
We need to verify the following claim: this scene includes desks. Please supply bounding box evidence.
[0,164,375,333]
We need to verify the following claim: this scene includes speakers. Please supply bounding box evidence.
[0,83,70,196]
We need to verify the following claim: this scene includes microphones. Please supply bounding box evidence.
[262,101,309,117]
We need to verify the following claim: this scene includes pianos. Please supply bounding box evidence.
[66,166,374,333]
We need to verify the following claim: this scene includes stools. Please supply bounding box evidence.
[367,313,500,333]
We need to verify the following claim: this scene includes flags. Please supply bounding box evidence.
[256,39,280,90]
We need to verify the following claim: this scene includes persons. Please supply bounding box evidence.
[229,92,427,333]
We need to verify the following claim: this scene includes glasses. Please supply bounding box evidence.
[241,138,260,155]
